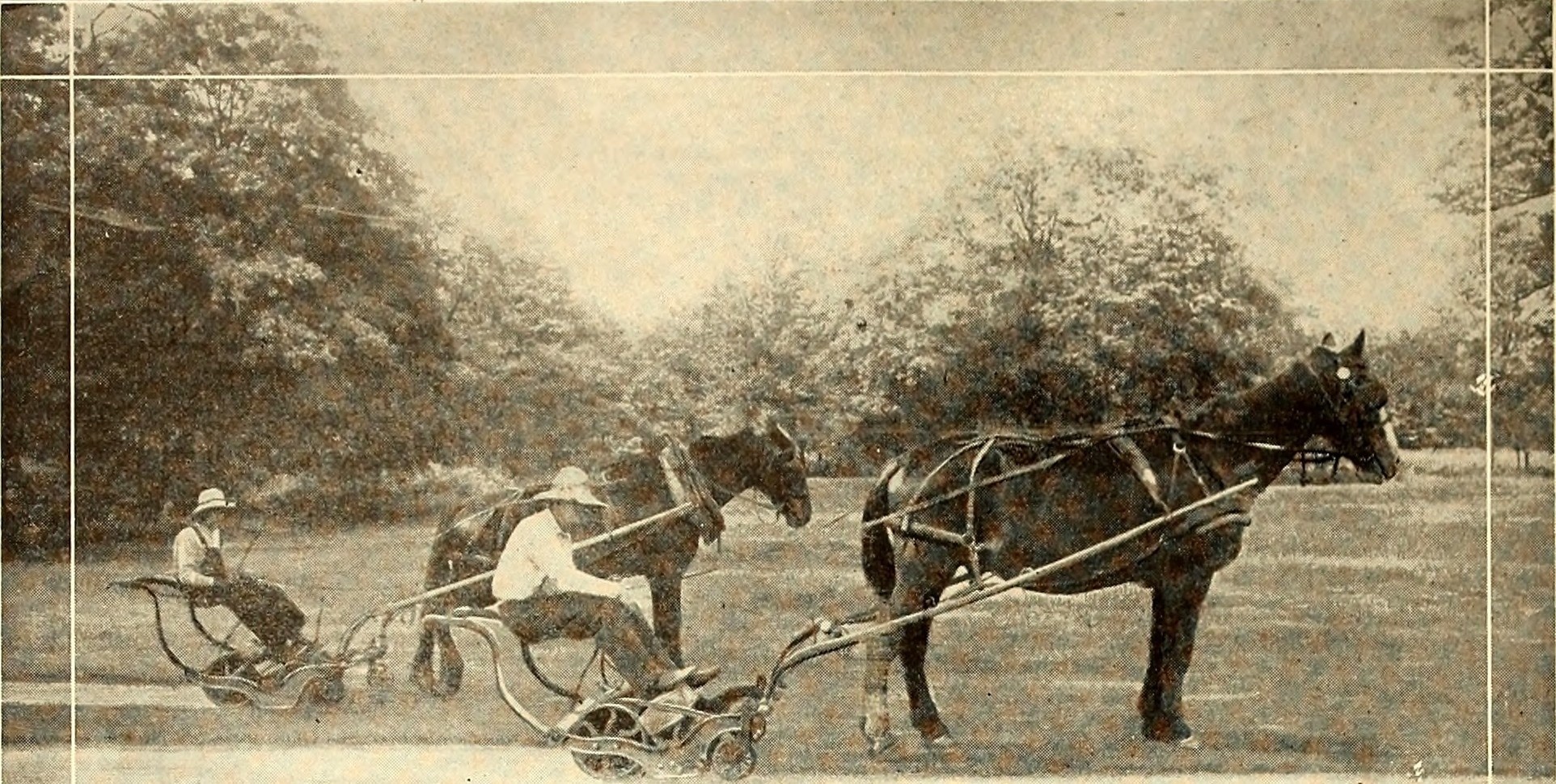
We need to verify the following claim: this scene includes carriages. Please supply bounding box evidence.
[424,310,1403,784]
[104,408,810,713]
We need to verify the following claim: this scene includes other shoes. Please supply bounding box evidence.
[683,664,720,688]
[643,664,696,698]
[268,636,337,665]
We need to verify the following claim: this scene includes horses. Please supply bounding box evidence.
[411,417,811,697]
[856,329,1403,763]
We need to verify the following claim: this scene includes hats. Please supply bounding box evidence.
[533,467,608,508]
[192,488,237,516]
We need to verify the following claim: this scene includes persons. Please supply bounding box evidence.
[490,465,722,700]
[171,487,267,615]
